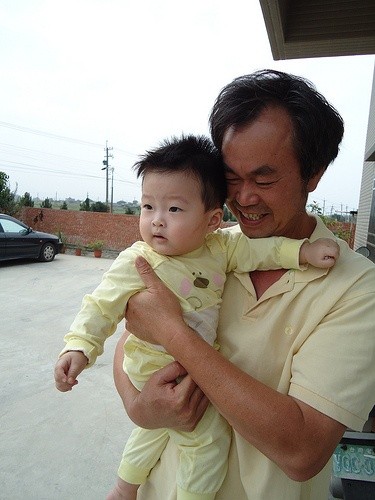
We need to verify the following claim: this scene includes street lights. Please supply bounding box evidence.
[110,167,114,213]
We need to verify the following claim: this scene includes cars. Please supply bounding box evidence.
[0,214,64,262]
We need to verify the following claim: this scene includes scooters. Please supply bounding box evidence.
[330,247,375,500]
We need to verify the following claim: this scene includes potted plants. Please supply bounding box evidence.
[74,239,84,256]
[54,224,68,254]
[87,240,106,258]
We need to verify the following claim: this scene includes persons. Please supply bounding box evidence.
[113,70,375,499]
[54,137,340,500]
